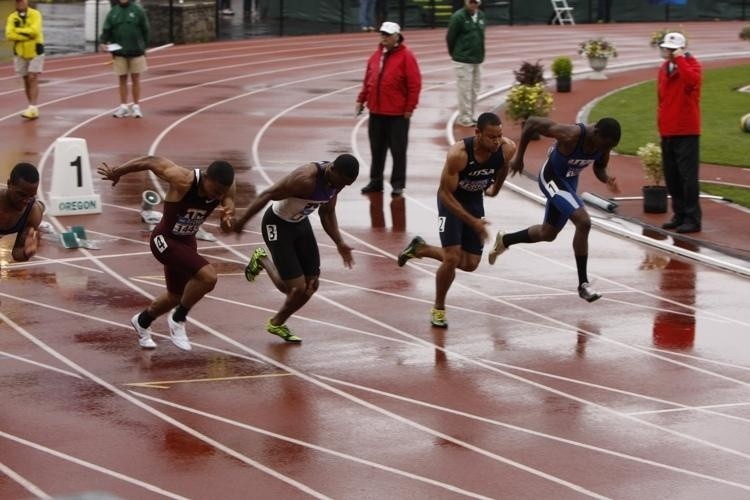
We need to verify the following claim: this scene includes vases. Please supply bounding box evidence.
[589,58,608,82]
[521,118,541,144]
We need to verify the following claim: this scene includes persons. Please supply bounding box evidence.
[5,1,45,119]
[657,32,704,234]
[98,155,235,351]
[0,162,45,263]
[356,22,421,195]
[447,0,486,127]
[359,0,377,32]
[398,113,516,328]
[100,0,151,119]
[230,154,359,343]
[489,115,621,303]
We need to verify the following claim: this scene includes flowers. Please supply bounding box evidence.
[577,36,620,61]
[506,84,554,121]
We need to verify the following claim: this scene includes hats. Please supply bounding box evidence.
[660,31,685,50]
[379,21,401,34]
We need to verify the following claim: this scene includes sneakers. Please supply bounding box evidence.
[457,116,475,127]
[245,247,268,281]
[431,307,448,328]
[20,105,39,118]
[113,103,130,117]
[473,119,477,124]
[265,316,303,342]
[577,283,602,302]
[132,104,142,118]
[167,309,192,351]
[398,235,425,267]
[488,231,509,264]
[130,311,157,348]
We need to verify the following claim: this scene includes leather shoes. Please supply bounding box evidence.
[676,219,701,233]
[392,186,403,195]
[662,216,682,229]
[362,181,384,192]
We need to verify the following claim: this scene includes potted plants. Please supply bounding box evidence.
[637,142,670,215]
[553,58,573,94]
